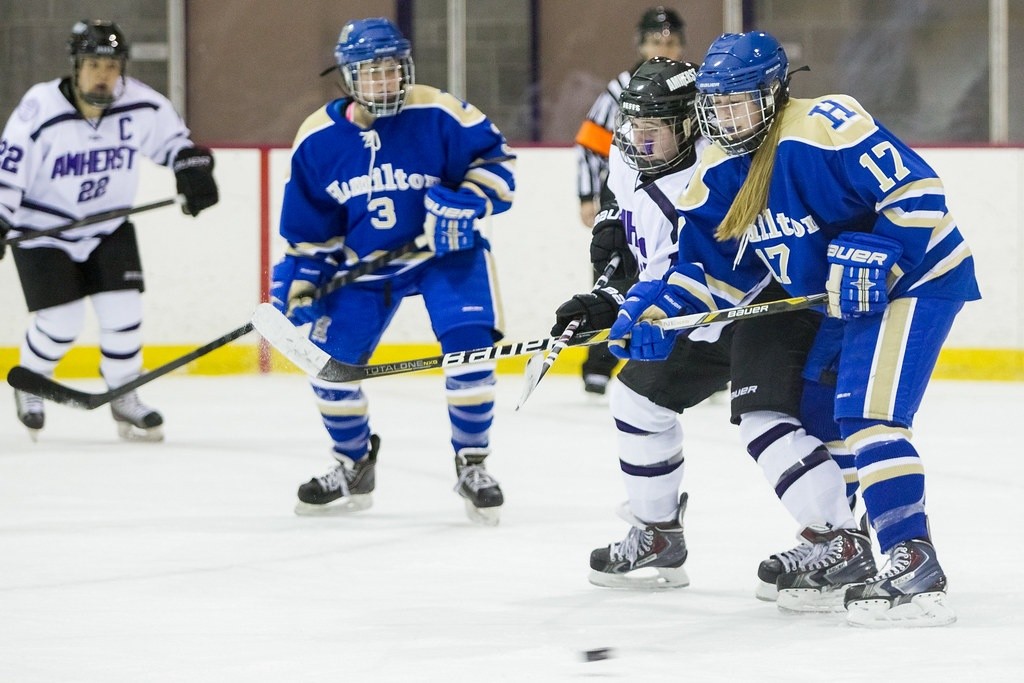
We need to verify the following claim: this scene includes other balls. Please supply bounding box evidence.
[583,647,613,663]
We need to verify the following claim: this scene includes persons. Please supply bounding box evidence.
[0,19,221,441]
[268,18,517,525]
[551,8,982,628]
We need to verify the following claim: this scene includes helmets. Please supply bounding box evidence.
[636,6,686,47]
[334,17,416,118]
[68,19,128,84]
[618,55,700,174]
[695,31,788,158]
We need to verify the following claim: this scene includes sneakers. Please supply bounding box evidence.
[843,514,955,626]
[776,509,878,615]
[294,433,380,515]
[754,493,857,602]
[454,447,503,527]
[587,490,689,590]
[98,365,165,441]
[13,387,44,441]
[607,278,692,361]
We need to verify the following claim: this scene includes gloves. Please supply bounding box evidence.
[589,207,636,280]
[551,285,631,346]
[171,145,220,217]
[421,185,486,258]
[824,231,906,320]
[269,253,332,329]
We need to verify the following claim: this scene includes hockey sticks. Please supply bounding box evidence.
[248,291,828,384]
[511,253,623,412]
[5,234,428,413]
[4,194,186,247]
